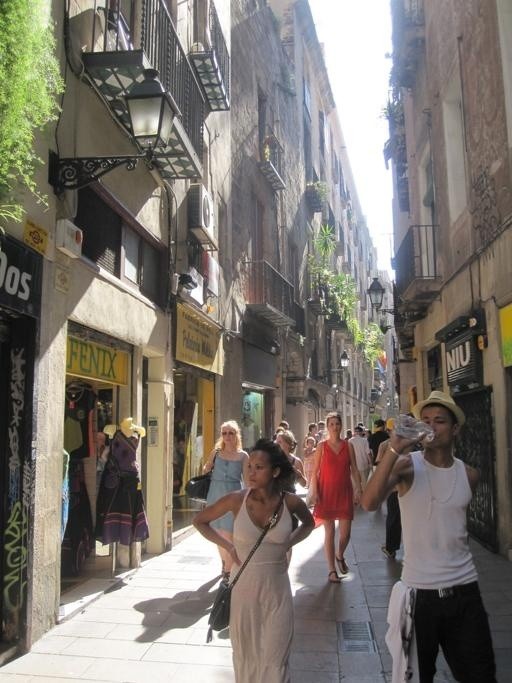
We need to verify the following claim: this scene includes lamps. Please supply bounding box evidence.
[324,352,349,378]
[48,69,182,194]
[366,278,398,315]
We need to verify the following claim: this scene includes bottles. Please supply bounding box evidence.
[396,414,436,443]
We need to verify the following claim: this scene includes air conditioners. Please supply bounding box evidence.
[189,183,213,244]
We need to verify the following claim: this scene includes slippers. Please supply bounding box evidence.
[336,556,348,574]
[329,571,341,583]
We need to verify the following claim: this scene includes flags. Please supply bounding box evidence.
[378,352,387,373]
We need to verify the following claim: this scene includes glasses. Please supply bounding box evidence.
[222,431,235,435]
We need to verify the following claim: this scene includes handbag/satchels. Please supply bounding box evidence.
[185,474,210,498]
[206,577,231,643]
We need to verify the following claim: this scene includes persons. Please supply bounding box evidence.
[192,438,315,683]
[359,391,497,683]
[272,411,421,586]
[202,421,250,573]
[92,416,149,545]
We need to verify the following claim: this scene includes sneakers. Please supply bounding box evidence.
[382,547,396,559]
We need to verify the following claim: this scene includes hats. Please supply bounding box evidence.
[374,420,386,432]
[386,418,397,430]
[412,391,466,426]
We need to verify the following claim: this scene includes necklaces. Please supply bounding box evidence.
[425,459,458,520]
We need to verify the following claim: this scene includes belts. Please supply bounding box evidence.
[418,582,476,599]
